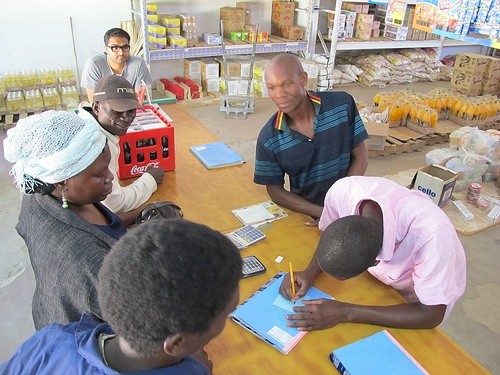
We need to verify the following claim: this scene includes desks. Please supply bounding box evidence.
[118,108,297,232]
[204,214,494,375]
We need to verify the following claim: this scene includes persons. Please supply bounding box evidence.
[3,109,154,332]
[278,176,467,331]
[80,27,152,105]
[75,74,164,214]
[0,218,244,375]
[253,54,368,226]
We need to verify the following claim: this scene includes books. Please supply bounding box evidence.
[228,270,337,355]
[189,142,246,170]
[231,200,288,227]
[330,329,430,375]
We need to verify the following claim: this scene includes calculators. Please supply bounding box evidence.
[224,225,267,251]
[238,255,266,279]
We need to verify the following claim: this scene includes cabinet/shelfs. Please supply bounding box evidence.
[129,0,315,103]
[310,0,479,87]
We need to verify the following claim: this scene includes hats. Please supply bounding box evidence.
[93,74,146,113]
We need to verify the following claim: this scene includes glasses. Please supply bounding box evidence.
[107,45,130,52]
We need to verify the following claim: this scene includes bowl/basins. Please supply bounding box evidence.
[476,197,490,210]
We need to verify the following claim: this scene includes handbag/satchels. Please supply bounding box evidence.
[136,201,183,226]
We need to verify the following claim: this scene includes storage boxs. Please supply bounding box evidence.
[118,105,176,179]
[145,0,500,150]
[409,164,458,209]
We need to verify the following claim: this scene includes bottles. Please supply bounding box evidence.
[356,88,500,129]
[0,68,79,110]
[123,135,168,164]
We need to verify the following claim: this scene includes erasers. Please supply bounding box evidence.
[274,255,284,264]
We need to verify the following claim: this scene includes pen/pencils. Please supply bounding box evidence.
[288,261,296,305]
